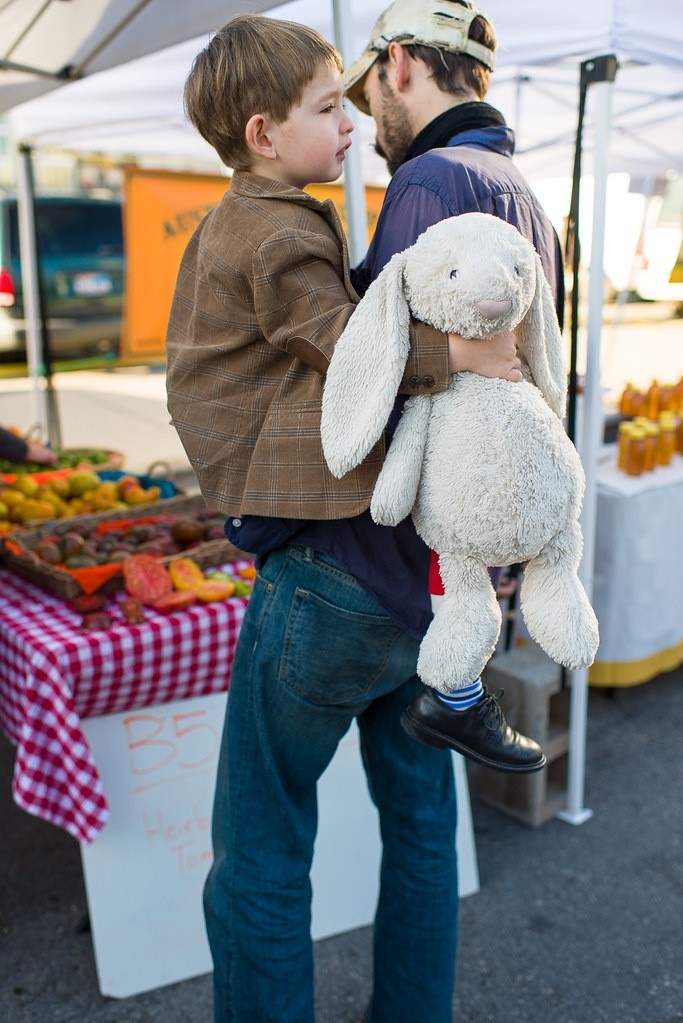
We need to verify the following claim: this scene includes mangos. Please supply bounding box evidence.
[0,472,161,529]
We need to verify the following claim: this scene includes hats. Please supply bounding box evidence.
[342,0,496,116]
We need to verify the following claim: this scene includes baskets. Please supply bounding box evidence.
[0,493,255,603]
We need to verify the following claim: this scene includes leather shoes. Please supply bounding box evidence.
[400,683,547,775]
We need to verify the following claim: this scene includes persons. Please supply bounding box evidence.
[203,0,563,1023]
[0,428,56,467]
[163,14,545,772]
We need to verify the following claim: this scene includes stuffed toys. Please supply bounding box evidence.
[318,212,598,688]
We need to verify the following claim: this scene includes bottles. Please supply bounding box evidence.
[616,376,683,475]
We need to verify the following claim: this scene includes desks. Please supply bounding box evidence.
[1,557,478,1000]
[578,446,683,688]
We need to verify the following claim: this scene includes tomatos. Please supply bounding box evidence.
[33,510,230,569]
[124,556,233,614]
